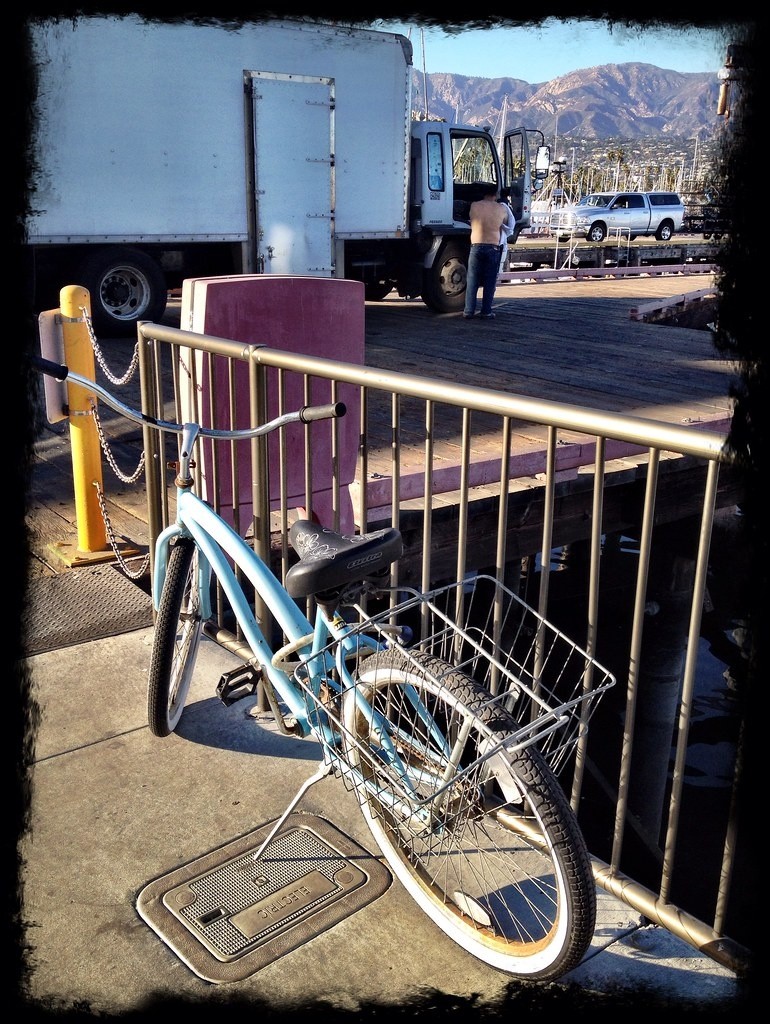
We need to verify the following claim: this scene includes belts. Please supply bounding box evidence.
[470,243,499,246]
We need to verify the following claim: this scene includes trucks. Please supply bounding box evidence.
[18,16,549,343]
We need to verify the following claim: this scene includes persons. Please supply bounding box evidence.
[462,184,514,318]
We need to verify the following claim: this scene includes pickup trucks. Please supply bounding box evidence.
[549,191,684,242]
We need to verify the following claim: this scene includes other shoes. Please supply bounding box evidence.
[462,312,473,318]
[482,313,496,319]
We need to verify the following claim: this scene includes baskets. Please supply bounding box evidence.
[294,574,617,867]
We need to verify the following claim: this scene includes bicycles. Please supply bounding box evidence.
[26,356,617,984]
